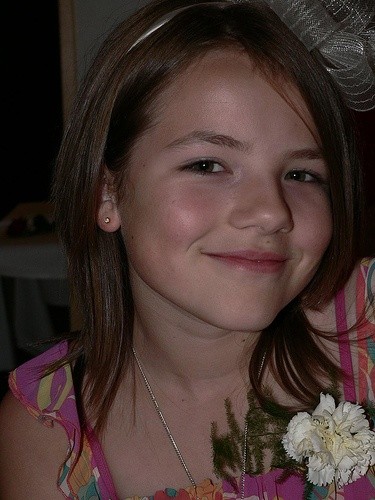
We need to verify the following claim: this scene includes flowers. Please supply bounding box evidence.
[281,393,374,500]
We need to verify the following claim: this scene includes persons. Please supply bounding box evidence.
[1,0,375,500]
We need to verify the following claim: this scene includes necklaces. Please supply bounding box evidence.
[128,339,269,500]
